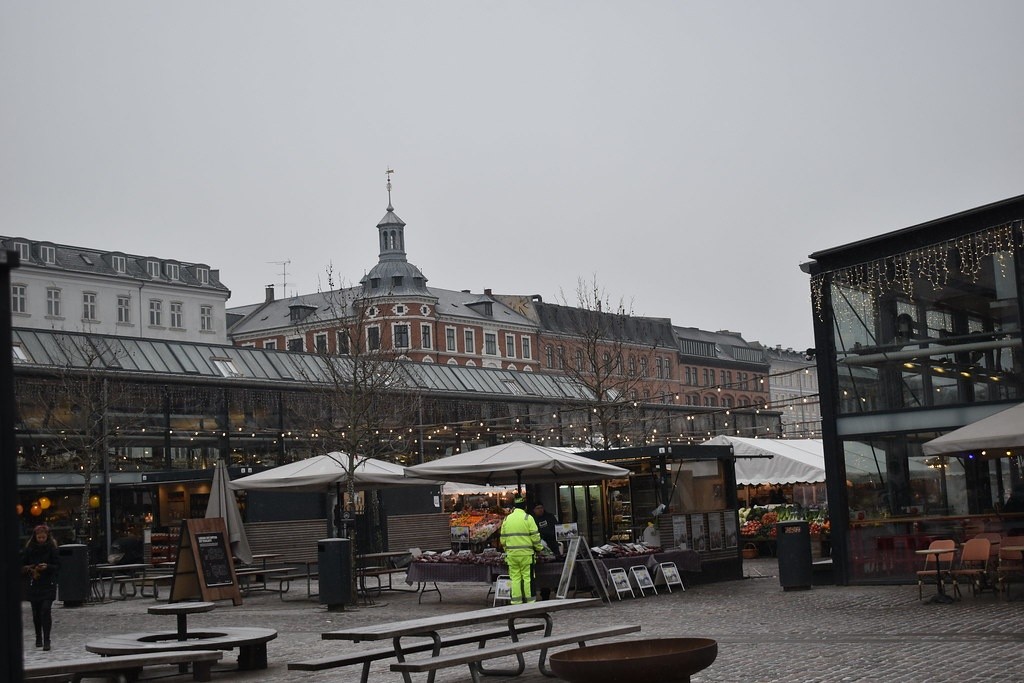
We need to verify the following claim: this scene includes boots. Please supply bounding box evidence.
[43,622,51,651]
[35,622,42,647]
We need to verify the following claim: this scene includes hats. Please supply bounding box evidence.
[532,502,543,510]
[513,494,525,508]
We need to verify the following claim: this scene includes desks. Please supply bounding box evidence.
[355,551,412,593]
[493,548,702,596]
[321,596,603,681]
[95,563,153,598]
[229,552,284,589]
[148,602,215,674]
[404,564,505,602]
[916,547,959,604]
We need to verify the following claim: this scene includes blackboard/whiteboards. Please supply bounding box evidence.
[194,532,233,588]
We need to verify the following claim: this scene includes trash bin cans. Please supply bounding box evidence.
[317,538,353,613]
[775,521,813,591]
[57,543,89,607]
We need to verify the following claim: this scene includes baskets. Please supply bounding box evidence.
[742,543,757,559]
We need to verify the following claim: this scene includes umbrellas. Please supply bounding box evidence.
[404,439,630,497]
[226,452,446,538]
[205,457,253,565]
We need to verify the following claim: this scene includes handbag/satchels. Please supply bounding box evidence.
[26,575,49,602]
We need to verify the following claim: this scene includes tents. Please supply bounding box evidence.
[667,434,938,511]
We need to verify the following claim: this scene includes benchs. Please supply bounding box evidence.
[84,627,279,676]
[286,622,545,683]
[24,649,222,683]
[86,567,422,605]
[388,623,641,682]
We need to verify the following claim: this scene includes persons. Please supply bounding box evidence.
[499,497,544,604]
[529,502,561,600]
[921,402,1024,461]
[21,525,60,651]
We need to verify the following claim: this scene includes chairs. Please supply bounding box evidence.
[974,533,1024,600]
[916,539,955,602]
[950,541,999,602]
